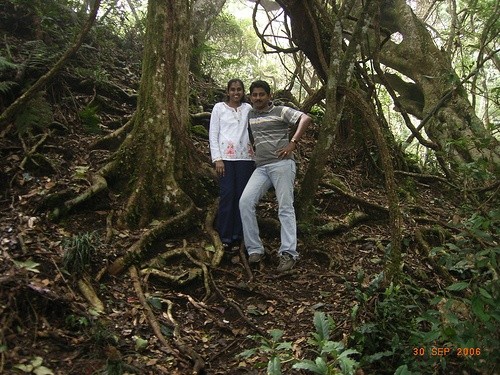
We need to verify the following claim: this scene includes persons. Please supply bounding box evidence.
[239,80,312,273]
[209,78,257,255]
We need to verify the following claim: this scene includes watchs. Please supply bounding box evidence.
[290,138,298,144]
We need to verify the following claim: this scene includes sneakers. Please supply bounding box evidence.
[247,253,266,264]
[276,251,296,272]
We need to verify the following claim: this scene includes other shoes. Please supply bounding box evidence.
[222,238,240,254]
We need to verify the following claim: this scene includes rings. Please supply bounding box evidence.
[284,151,287,154]
